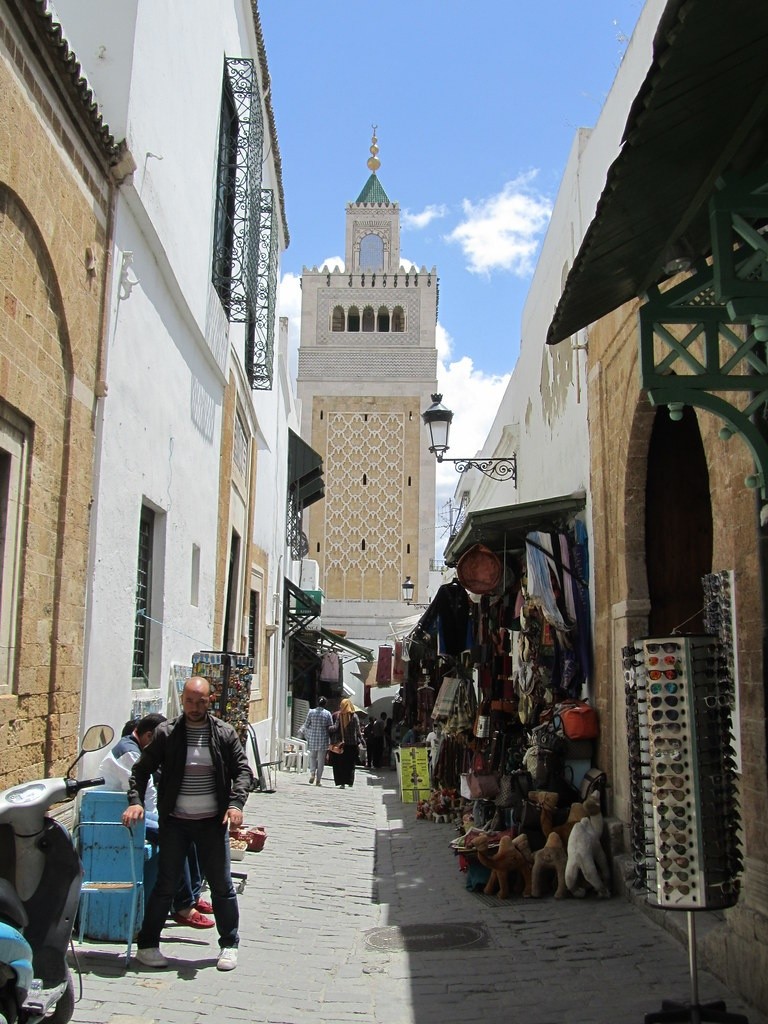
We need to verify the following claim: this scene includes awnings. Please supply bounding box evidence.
[282,576,321,646]
[289,627,376,684]
[443,491,586,569]
[389,578,458,644]
[547,0,767,526]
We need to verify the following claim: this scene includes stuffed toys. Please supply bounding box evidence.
[472,791,610,899]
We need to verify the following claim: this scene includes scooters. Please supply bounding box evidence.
[0,724,116,1024]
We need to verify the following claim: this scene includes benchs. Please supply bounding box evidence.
[77,791,161,944]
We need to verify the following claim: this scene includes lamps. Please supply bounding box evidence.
[401,576,432,605]
[420,392,518,490]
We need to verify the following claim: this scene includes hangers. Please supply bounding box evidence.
[450,572,461,585]
[378,637,391,648]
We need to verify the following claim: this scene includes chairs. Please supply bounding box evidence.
[73,822,144,967]
[280,736,310,773]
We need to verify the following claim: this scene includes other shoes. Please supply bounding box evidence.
[309,777,314,783]
[316,782,321,786]
[194,899,213,913]
[174,910,214,928]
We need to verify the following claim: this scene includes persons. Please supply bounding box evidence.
[121,720,141,738]
[327,699,366,789]
[304,696,334,786]
[121,676,253,971]
[94,713,215,928]
[363,712,392,769]
[402,720,446,792]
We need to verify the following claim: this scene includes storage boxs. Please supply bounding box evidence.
[460,773,499,800]
[229,844,248,860]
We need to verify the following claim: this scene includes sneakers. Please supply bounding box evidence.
[136,947,169,966]
[217,948,238,970]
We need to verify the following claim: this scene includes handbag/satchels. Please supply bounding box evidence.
[329,742,344,754]
[459,589,608,841]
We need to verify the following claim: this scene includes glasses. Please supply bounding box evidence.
[619,570,746,902]
